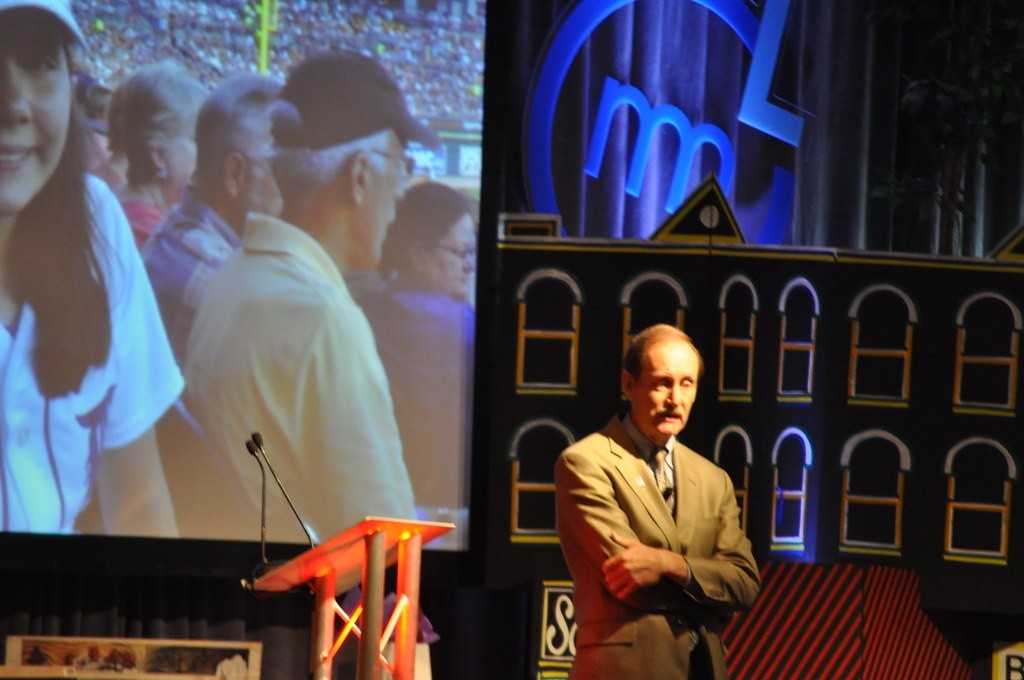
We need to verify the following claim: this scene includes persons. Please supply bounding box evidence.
[0,1,485,554]
[555,324,759,680]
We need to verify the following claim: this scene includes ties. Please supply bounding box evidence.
[650,448,675,515]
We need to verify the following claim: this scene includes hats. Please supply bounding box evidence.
[0,0,89,52]
[268,49,441,151]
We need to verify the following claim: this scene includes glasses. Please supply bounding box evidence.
[373,150,416,173]
[437,243,474,258]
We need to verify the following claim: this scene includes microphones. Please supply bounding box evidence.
[244,430,319,572]
[665,487,673,497]
